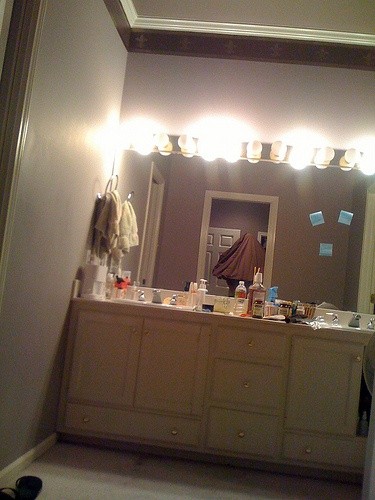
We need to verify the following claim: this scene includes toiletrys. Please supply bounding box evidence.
[187,278,208,309]
[247,272,267,317]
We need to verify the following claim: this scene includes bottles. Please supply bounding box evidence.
[234,280,246,316]
[249,273,266,319]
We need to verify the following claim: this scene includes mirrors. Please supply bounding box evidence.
[106,132,375,316]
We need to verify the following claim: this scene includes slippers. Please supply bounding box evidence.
[0,488,18,500]
[16,475,42,500]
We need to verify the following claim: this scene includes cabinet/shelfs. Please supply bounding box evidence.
[57,301,372,483]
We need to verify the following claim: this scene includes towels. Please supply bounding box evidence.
[108,199,139,275]
[91,190,122,261]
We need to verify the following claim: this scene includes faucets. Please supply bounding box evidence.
[347,313,361,328]
[151,289,161,303]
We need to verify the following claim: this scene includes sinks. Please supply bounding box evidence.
[110,298,194,310]
